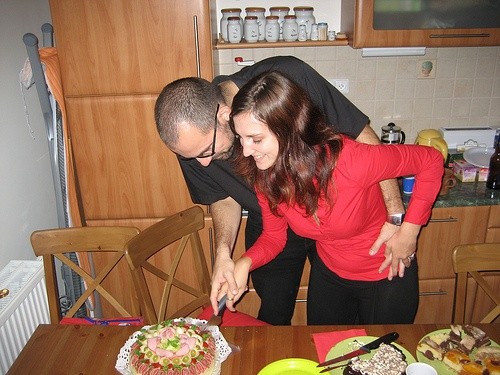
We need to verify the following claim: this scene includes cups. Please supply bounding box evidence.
[402,176,415,195]
[439,167,456,196]
[405,362,438,375]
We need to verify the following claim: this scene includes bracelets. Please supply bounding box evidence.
[387,213,405,225]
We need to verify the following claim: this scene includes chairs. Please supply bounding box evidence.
[30,226,143,326]
[453,245,500,325]
[124,206,273,326]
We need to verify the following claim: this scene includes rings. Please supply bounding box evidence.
[0,289,9,298]
[409,254,415,258]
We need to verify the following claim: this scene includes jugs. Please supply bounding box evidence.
[381,123,406,145]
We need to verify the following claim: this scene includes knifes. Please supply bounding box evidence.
[317,332,401,368]
[203,295,226,331]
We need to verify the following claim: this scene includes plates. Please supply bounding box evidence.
[463,147,495,168]
[324,335,417,375]
[416,329,500,375]
[256,358,331,375]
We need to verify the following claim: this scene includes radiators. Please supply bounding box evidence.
[0,256,51,375]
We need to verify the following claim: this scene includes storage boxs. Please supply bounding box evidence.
[445,150,465,168]
[476,167,489,182]
[454,160,477,182]
[439,126,495,148]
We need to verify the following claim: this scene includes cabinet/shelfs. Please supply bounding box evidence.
[47,0,500,329]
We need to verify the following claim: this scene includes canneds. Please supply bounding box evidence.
[317,23,327,40]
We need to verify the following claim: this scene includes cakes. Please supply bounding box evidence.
[127,320,216,375]
[342,341,408,375]
[417,323,500,375]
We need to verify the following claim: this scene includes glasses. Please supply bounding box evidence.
[177,104,219,162]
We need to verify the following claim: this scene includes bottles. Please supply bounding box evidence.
[329,31,336,41]
[220,6,318,44]
[486,130,500,189]
[318,23,328,41]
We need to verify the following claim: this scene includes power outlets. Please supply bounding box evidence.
[334,79,350,94]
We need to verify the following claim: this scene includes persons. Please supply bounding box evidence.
[154,56,444,324]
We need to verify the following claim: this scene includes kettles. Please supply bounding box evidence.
[413,128,448,166]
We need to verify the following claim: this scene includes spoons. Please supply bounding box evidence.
[320,357,360,373]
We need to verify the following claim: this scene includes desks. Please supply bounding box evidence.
[7,324,500,375]
